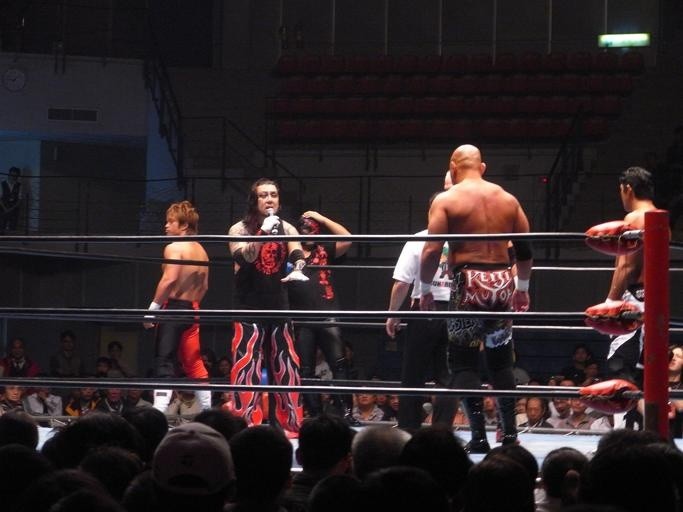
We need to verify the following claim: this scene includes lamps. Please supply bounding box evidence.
[597,33,650,48]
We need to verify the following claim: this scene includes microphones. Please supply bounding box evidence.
[267,208,278,234]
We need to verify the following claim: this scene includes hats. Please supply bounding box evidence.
[148,422,238,494]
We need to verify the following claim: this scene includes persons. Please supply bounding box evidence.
[25,388,63,418]
[59,355,83,377]
[99,388,129,415]
[202,353,209,367]
[575,359,600,385]
[228,180,306,440]
[667,346,683,440]
[344,335,380,379]
[315,344,333,400]
[419,144,533,452]
[384,395,400,421]
[48,333,74,373]
[560,379,575,386]
[96,356,112,375]
[353,392,384,422]
[520,397,554,429]
[228,426,409,512]
[0,166,24,235]
[0,418,126,512]
[606,166,672,416]
[130,416,228,512]
[66,388,97,417]
[558,399,596,430]
[443,170,516,442]
[369,329,408,380]
[528,379,541,386]
[409,433,683,511]
[566,343,592,381]
[144,201,212,414]
[128,390,152,412]
[1,386,24,416]
[386,192,461,436]
[549,378,556,385]
[109,340,127,369]
[283,211,353,423]
[219,358,231,375]
[0,337,40,377]
